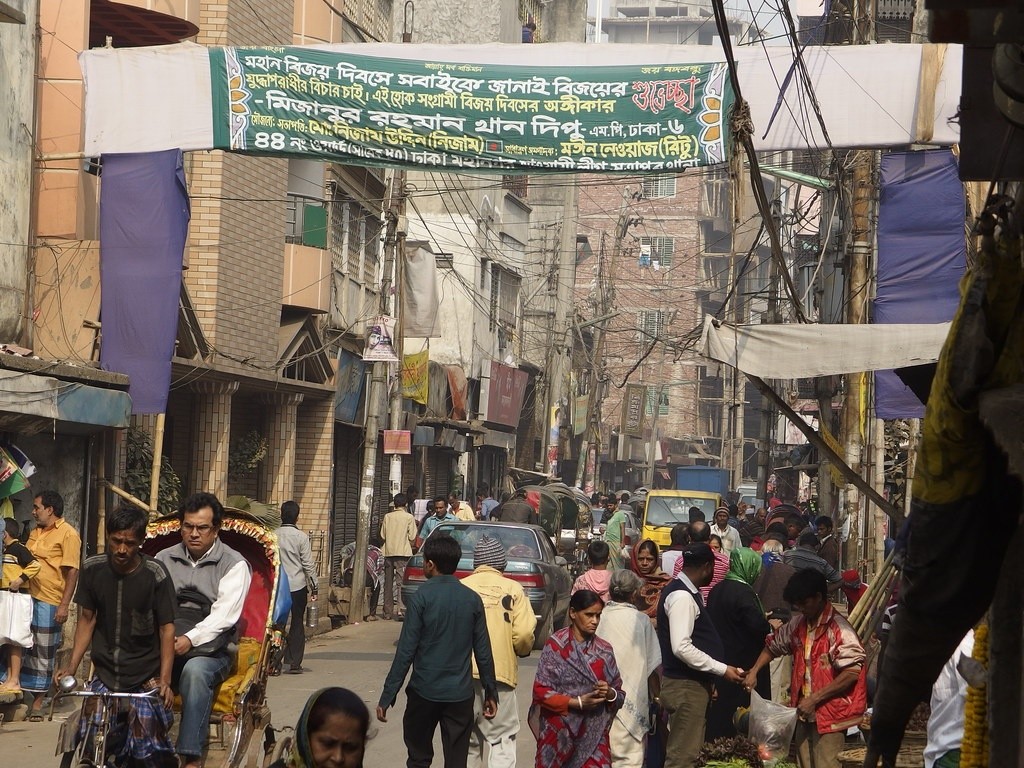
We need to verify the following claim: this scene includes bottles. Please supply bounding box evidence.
[574,541,580,561]
[306,599,319,628]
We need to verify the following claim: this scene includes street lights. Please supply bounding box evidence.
[740,157,874,574]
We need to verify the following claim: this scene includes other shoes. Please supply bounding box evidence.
[383,614,391,619]
[289,665,301,674]
[270,662,282,676]
[397,611,405,620]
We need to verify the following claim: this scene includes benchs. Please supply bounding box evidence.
[507,545,537,559]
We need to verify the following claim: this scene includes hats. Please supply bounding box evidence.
[682,543,723,565]
[473,534,508,571]
[713,507,731,518]
[745,508,755,515]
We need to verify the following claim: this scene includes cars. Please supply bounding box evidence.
[734,486,771,514]
[401,521,577,650]
[559,507,643,568]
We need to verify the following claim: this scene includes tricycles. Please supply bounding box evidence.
[46,505,284,768]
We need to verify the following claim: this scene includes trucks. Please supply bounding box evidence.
[641,489,724,559]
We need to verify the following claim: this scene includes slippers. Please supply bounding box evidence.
[0,683,22,695]
[29,709,44,722]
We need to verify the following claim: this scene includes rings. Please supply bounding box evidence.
[747,686,750,690]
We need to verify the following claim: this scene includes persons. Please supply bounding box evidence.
[657,543,745,768]
[589,490,646,570]
[153,492,254,768]
[595,570,662,767]
[377,536,501,768]
[458,534,536,768]
[54,504,182,768]
[497,488,537,525]
[923,628,975,768]
[662,499,869,768]
[0,490,83,722]
[630,537,673,634]
[272,499,319,674]
[571,542,614,605]
[380,486,499,621]
[269,687,380,768]
[0,517,41,694]
[527,589,627,768]
[862,244,1024,768]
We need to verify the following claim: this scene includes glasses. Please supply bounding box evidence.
[181,523,216,534]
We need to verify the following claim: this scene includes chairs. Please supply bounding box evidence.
[173,637,264,715]
[449,529,470,548]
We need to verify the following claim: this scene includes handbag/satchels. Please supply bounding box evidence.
[0,590,34,650]
[748,687,800,767]
[169,589,238,656]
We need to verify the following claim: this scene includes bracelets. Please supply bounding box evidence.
[606,687,618,702]
[577,694,583,710]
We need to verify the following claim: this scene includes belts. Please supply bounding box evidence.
[1,588,27,593]
